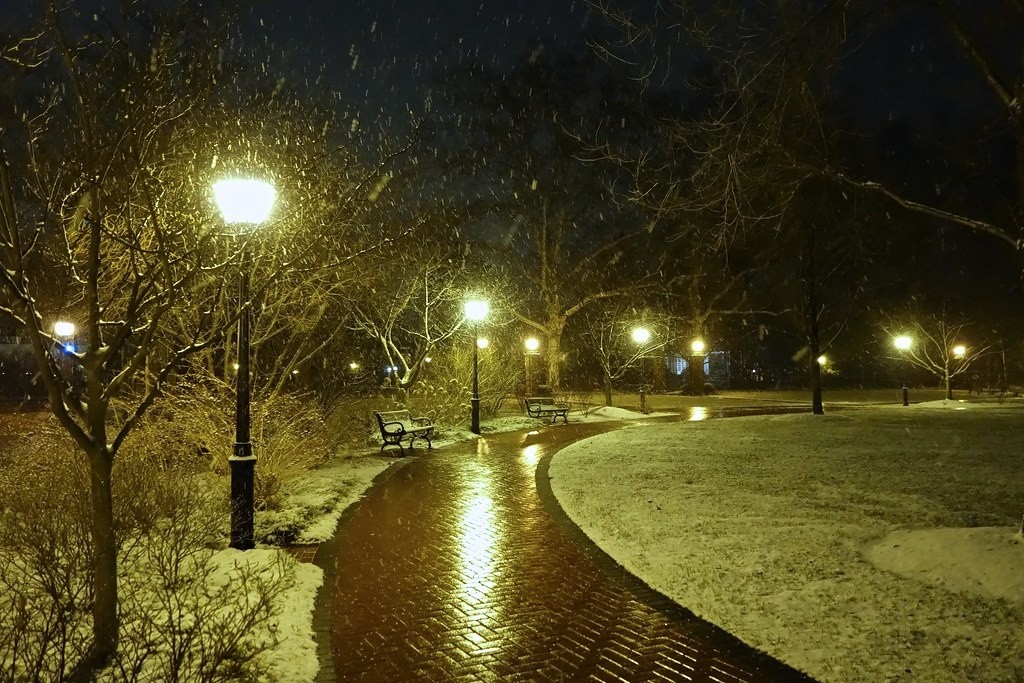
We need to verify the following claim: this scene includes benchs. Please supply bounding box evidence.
[524,397,569,425]
[375,407,434,458]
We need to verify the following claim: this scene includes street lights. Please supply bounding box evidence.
[631,328,650,413]
[463,299,490,435]
[213,178,279,549]
[894,336,913,407]
